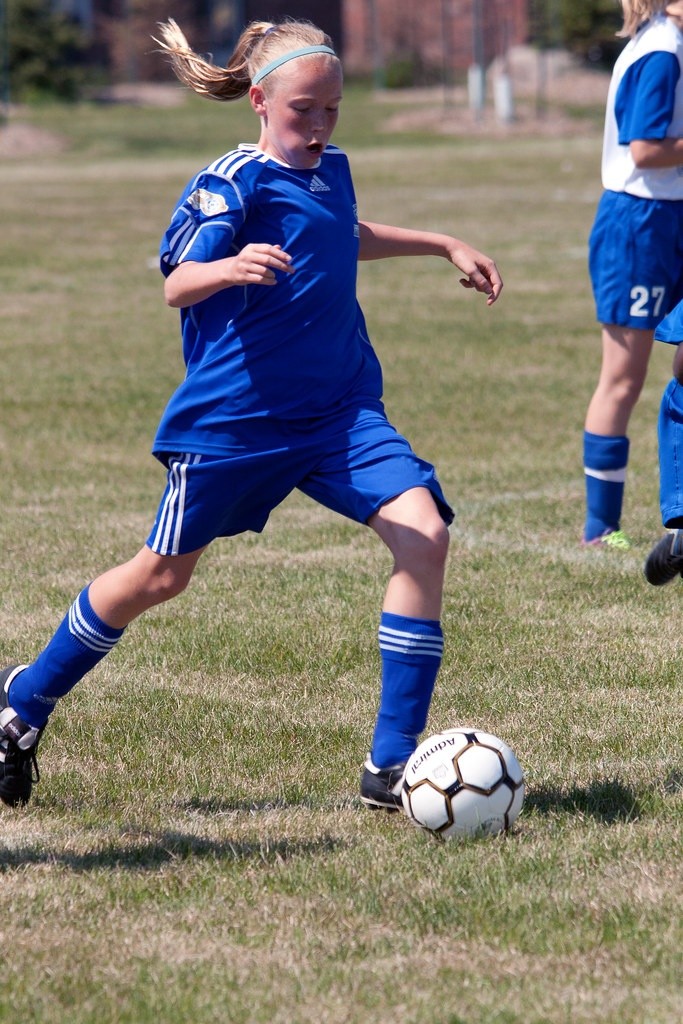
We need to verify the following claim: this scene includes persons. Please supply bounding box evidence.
[0,15,508,818]
[642,293,683,590]
[572,1,683,553]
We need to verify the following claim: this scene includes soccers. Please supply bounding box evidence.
[399,725,525,847]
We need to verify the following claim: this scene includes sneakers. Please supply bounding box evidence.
[0,663,48,808]
[643,529,682,585]
[358,750,405,813]
[581,525,633,550]
